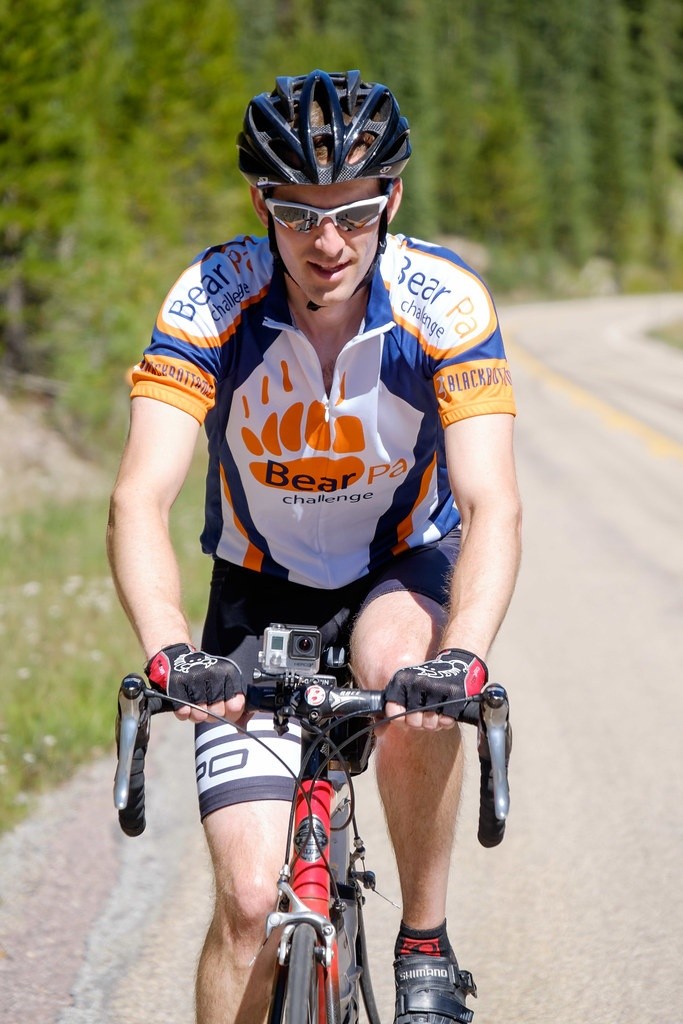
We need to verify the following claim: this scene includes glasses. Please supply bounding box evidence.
[262,178,394,233]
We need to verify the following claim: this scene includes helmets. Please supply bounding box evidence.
[236,69,412,187]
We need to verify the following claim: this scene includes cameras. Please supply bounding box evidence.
[259,624,323,679]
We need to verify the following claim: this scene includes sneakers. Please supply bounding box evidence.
[394,946,476,1024]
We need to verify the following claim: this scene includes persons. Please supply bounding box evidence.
[102,69,525,1022]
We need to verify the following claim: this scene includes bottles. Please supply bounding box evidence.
[329,902,352,1008]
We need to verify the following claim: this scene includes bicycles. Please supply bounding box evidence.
[99,617,516,1024]
